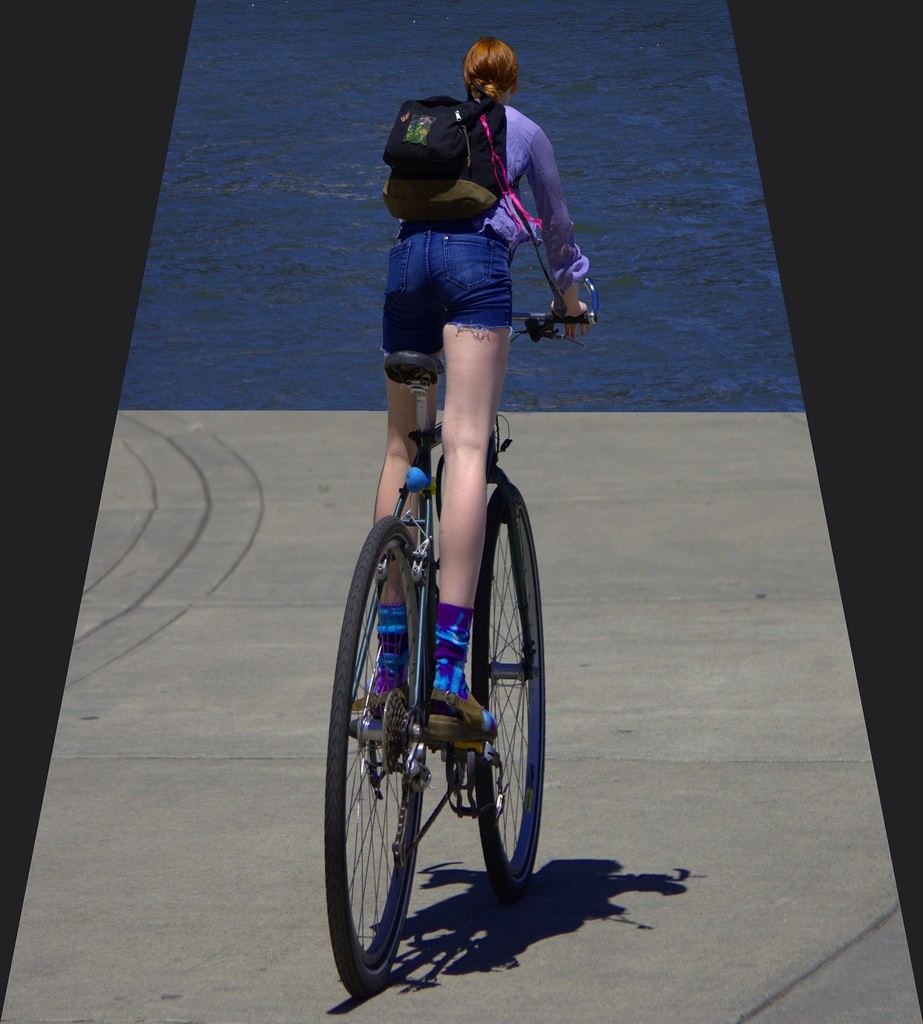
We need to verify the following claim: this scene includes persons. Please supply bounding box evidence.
[367,38,594,743]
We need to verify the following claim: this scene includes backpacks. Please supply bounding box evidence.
[381,95,507,221]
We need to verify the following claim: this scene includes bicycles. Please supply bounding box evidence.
[320,304,599,1001]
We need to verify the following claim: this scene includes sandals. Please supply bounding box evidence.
[350,687,499,743]
